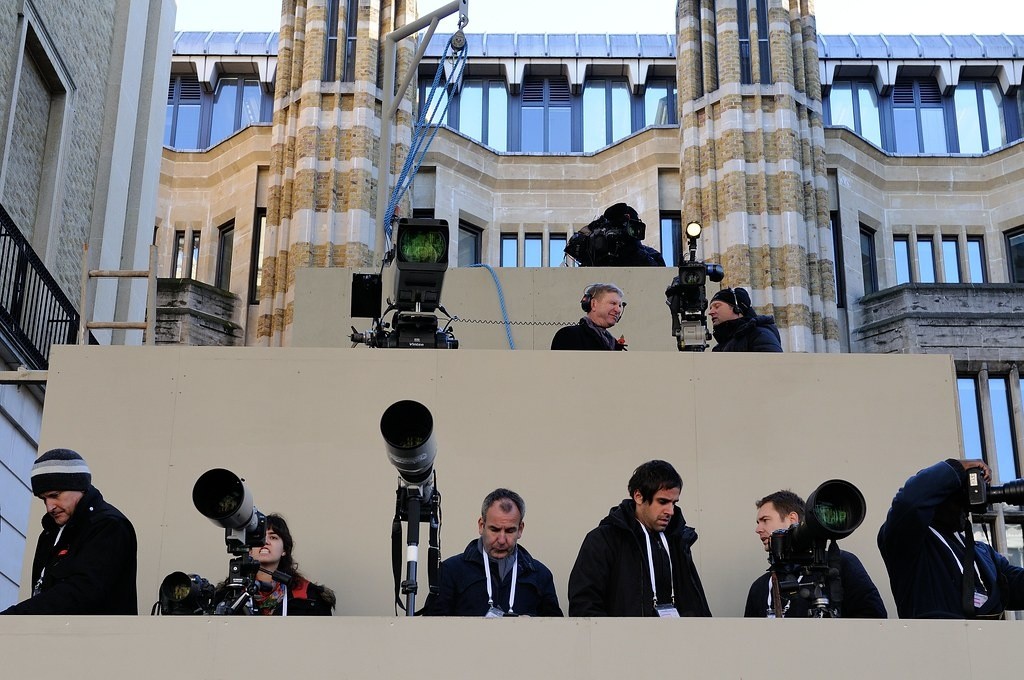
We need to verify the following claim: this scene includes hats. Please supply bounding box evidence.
[711,288,751,313]
[31,448,93,497]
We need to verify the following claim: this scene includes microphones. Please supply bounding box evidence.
[617,302,627,323]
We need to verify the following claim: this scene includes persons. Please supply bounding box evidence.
[877,459,1024,620]
[209,512,336,617]
[568,460,713,617]
[422,488,564,617]
[708,287,783,353]
[550,283,627,351]
[578,203,666,267]
[0,449,139,616]
[743,489,888,619]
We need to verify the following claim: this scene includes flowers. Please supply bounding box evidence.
[616,333,629,351]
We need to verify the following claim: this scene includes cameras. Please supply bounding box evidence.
[967,468,1024,513]
[195,468,268,553]
[381,400,438,520]
[768,479,867,574]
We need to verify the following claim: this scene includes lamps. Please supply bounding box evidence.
[684,221,703,261]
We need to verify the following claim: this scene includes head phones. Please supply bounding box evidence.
[581,283,604,312]
[732,288,741,314]
